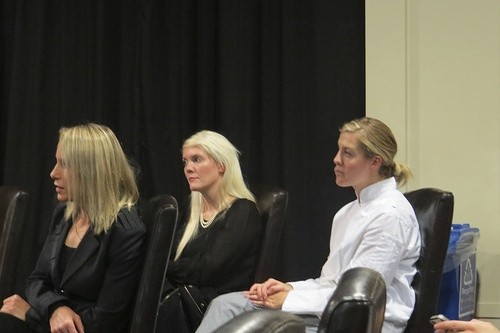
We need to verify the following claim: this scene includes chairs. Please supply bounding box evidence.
[317,268,386,333]
[181,188,289,289]
[403,188,454,333]
[48,193,178,333]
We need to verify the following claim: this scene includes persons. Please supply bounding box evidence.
[0,123,147,333]
[196,116,421,333]
[154,130,260,333]
[433,319,500,333]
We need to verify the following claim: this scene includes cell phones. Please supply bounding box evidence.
[429,314,449,325]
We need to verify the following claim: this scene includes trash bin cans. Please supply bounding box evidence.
[437,225,481,322]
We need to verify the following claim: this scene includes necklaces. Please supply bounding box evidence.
[74,223,82,240]
[199,189,233,229]
